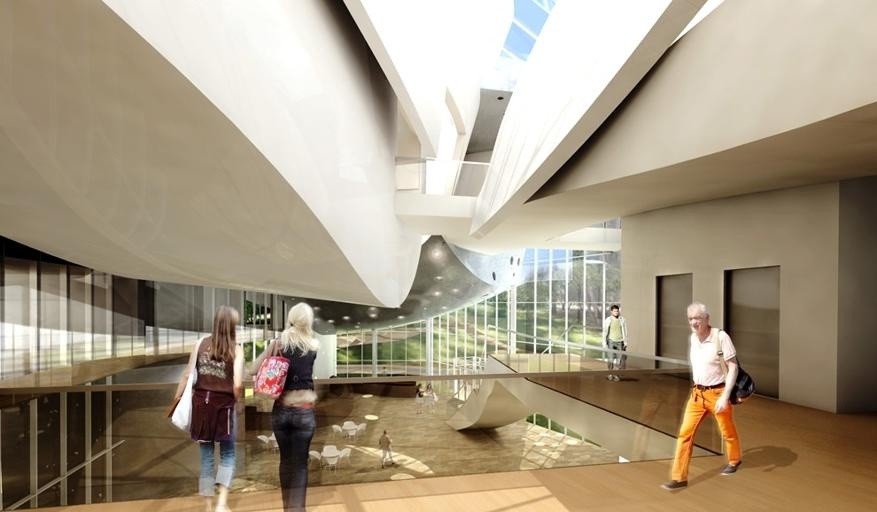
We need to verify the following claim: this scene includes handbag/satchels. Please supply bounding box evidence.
[254,339,291,398]
[169,390,193,432]
[723,371,756,404]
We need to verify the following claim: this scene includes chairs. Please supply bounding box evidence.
[256,432,279,454]
[309,420,367,474]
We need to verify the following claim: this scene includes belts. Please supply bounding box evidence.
[691,384,725,391]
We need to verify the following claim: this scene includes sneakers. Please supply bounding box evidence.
[720,461,742,475]
[661,479,688,491]
[607,375,622,381]
